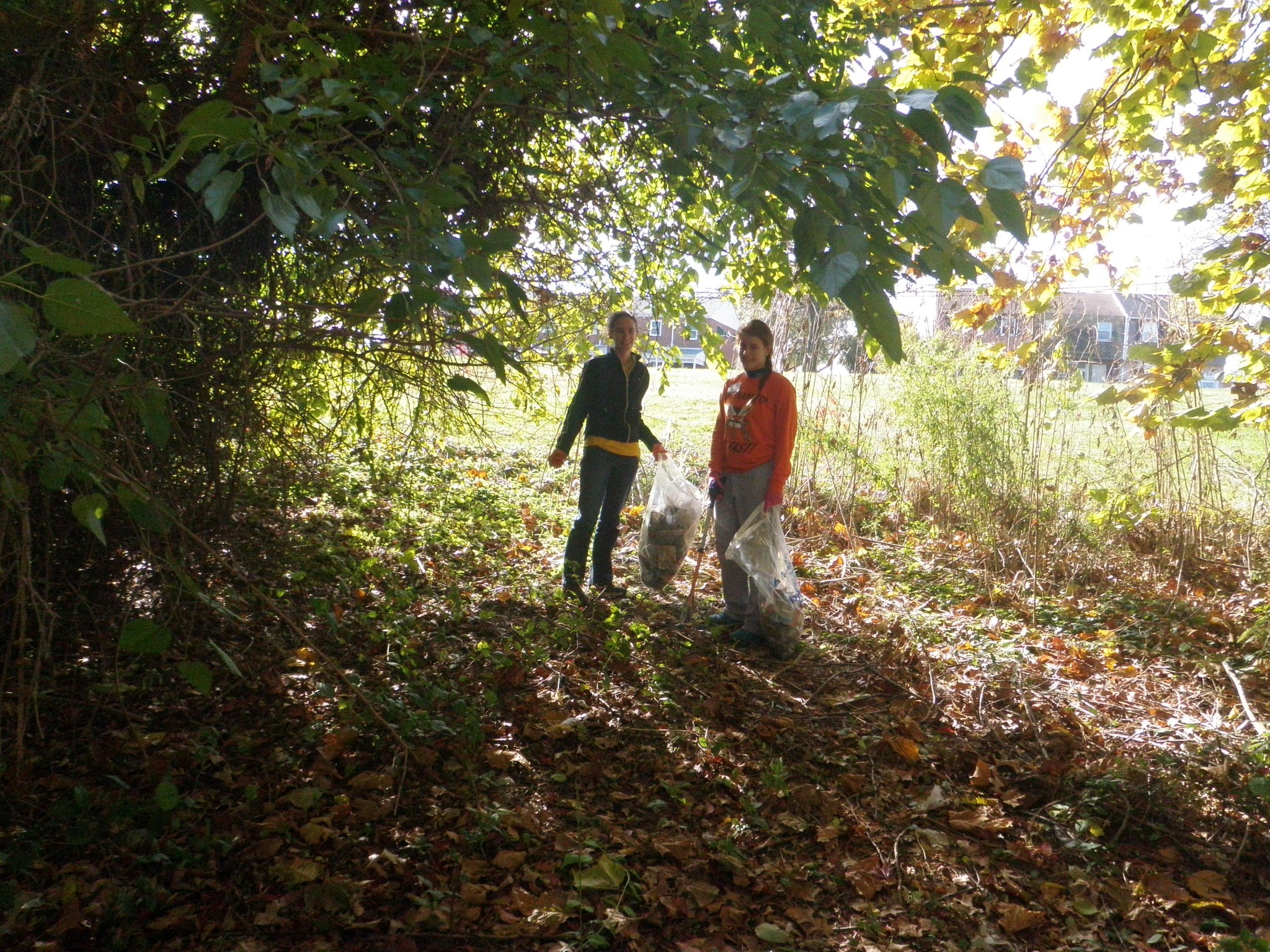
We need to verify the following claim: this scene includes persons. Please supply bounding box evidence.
[707,318,798,643]
[548,311,667,615]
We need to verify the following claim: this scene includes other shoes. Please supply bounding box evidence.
[588,583,627,595]
[562,584,586,599]
[721,629,759,643]
[710,612,744,627]
